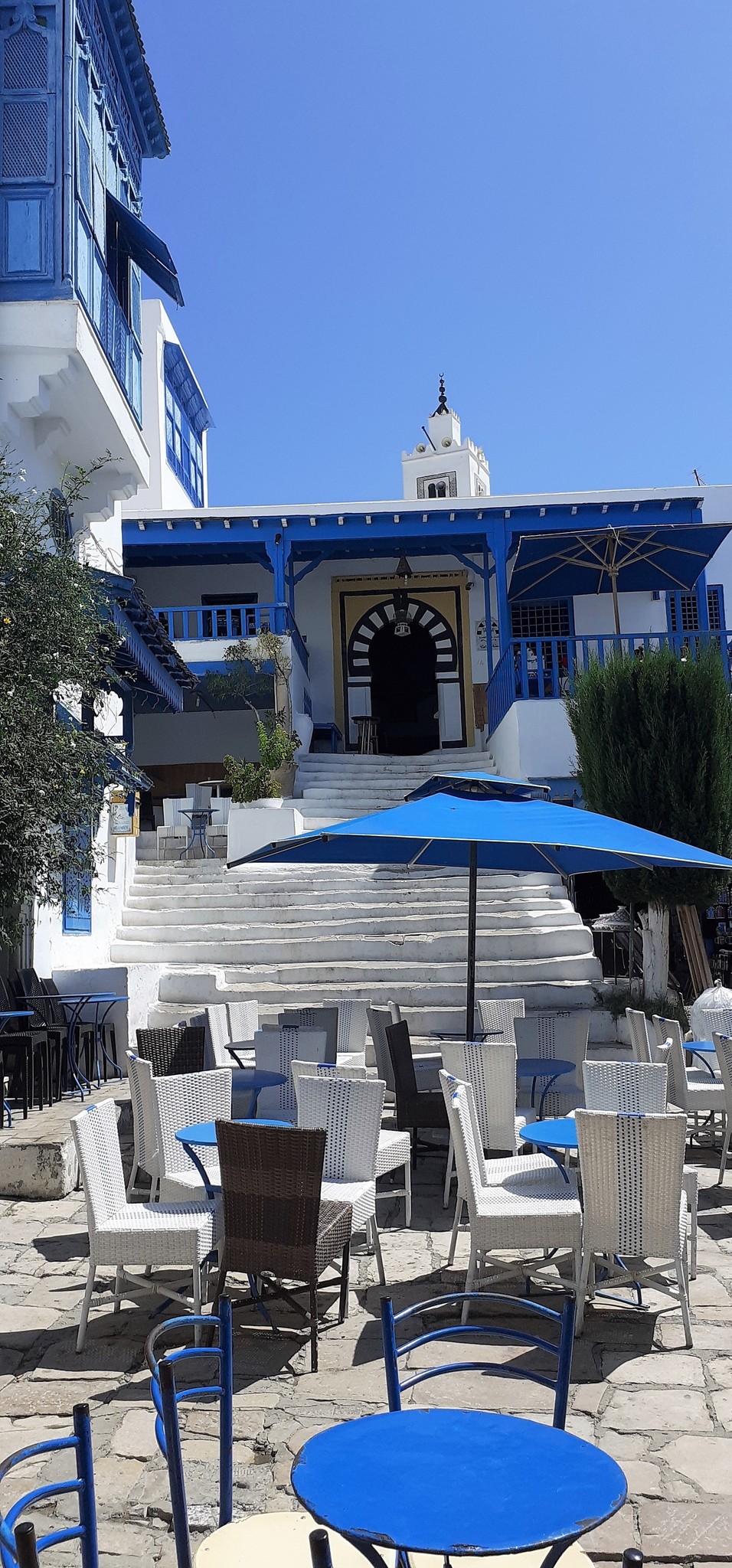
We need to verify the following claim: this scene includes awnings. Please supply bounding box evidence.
[106,187,185,307]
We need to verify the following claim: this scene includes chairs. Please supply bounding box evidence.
[156,798,195,859]
[0,968,732,1568]
[206,796,234,859]
[353,720,379,754]
[185,783,210,828]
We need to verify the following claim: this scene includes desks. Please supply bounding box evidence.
[198,779,226,799]
[294,1409,628,1568]
[430,1028,504,1042]
[11,993,116,1102]
[517,1058,576,1183]
[149,1118,313,1335]
[177,809,220,860]
[229,1068,288,1119]
[351,716,380,753]
[222,1039,255,1070]
[313,723,342,753]
[681,1041,722,1150]
[0,1011,33,1125]
[519,1116,647,1310]
[62,997,130,1086]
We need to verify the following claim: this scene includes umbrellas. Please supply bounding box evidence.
[507,522,732,667]
[226,771,732,1042]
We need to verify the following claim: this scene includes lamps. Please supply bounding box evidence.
[275,533,282,544]
[396,556,412,584]
